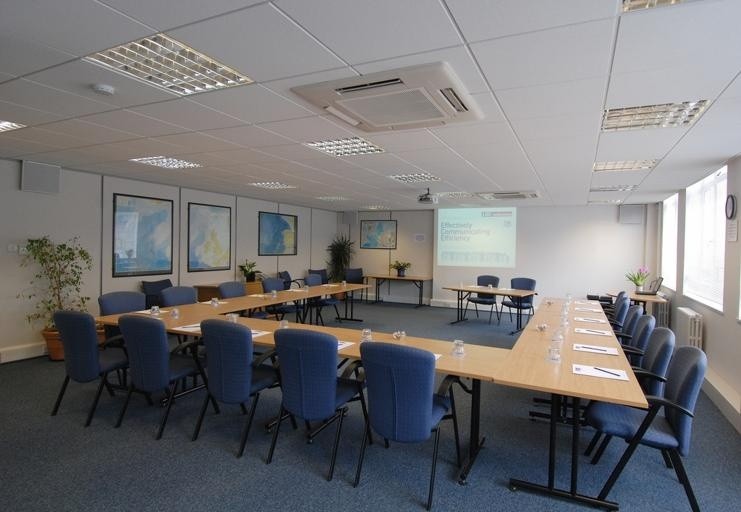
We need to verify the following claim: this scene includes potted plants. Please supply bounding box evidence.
[389,260,411,277]
[240,260,261,283]
[324,232,354,300]
[15,233,94,360]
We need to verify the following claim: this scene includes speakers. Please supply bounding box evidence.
[21,160,61,196]
[342,210,358,224]
[619,204,645,223]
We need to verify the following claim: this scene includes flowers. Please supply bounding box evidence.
[625,265,650,285]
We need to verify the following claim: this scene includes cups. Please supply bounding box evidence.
[171,309,179,320]
[342,281,346,286]
[280,320,289,329]
[304,286,309,293]
[545,298,553,306]
[392,330,407,341]
[536,321,548,332]
[272,290,277,297]
[225,314,239,323]
[211,298,219,307]
[549,293,572,360]
[359,328,372,343]
[488,285,492,290]
[452,339,464,356]
[151,306,160,316]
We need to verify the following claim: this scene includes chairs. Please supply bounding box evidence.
[97,269,342,387]
[497,277,536,327]
[581,291,707,511]
[463,275,499,325]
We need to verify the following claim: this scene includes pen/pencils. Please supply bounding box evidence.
[587,329,605,334]
[582,346,607,352]
[183,326,200,328]
[594,368,620,376]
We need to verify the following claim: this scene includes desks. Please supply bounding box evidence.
[441,284,538,335]
[95,285,647,510]
[369,274,433,308]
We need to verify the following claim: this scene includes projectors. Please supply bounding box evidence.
[417,194,438,204]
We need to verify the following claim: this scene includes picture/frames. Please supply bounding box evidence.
[111,192,174,277]
[188,201,231,271]
[359,219,398,249]
[258,211,297,256]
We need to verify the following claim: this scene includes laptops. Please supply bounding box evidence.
[635,277,664,295]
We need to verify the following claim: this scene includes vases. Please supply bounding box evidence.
[636,285,643,293]
